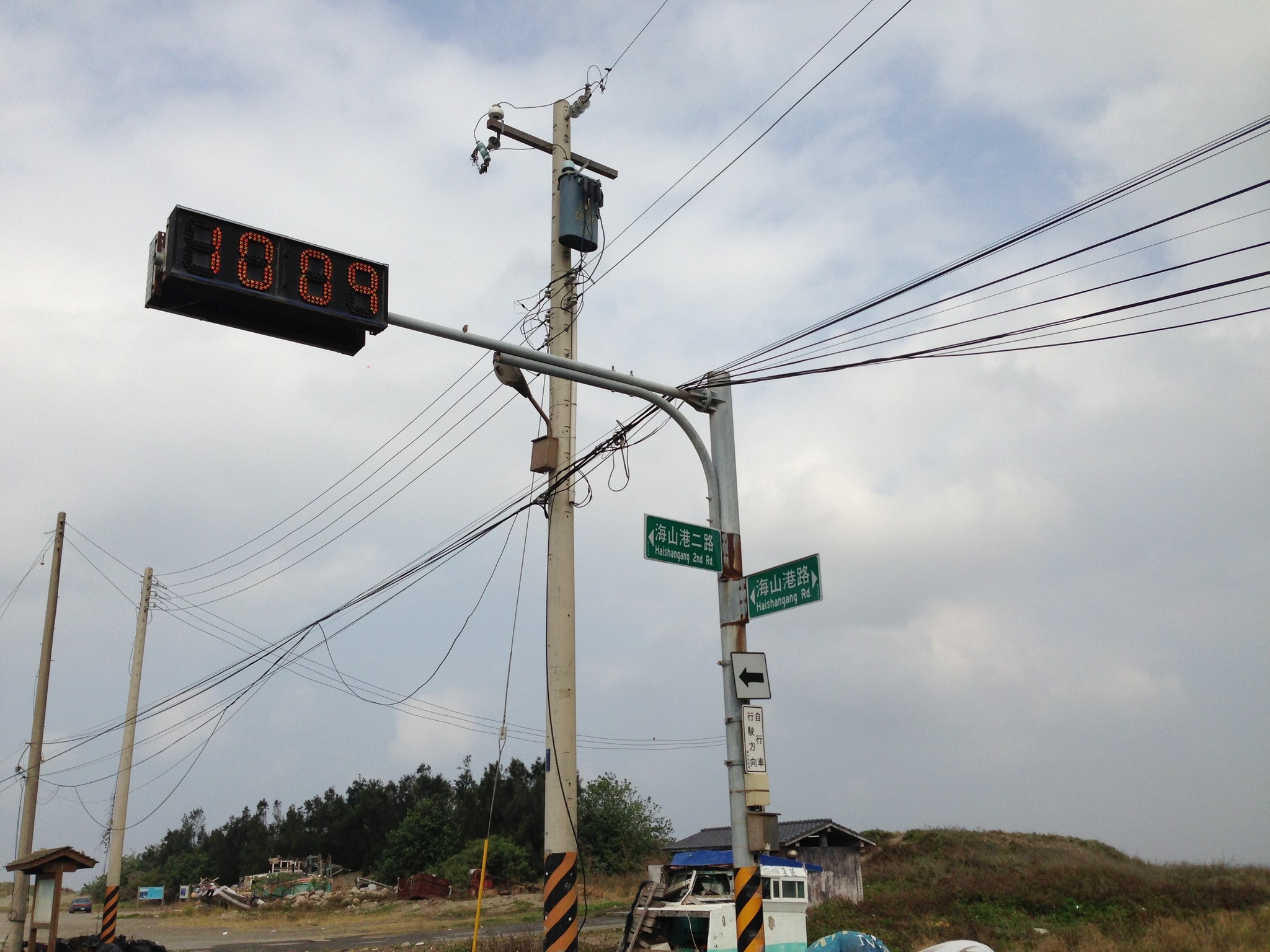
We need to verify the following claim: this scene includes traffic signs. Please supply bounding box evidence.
[747,554,824,620]
[644,514,723,574]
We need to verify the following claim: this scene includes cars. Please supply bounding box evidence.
[68,898,92,913]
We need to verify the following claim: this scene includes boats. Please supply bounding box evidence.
[239,854,351,898]
